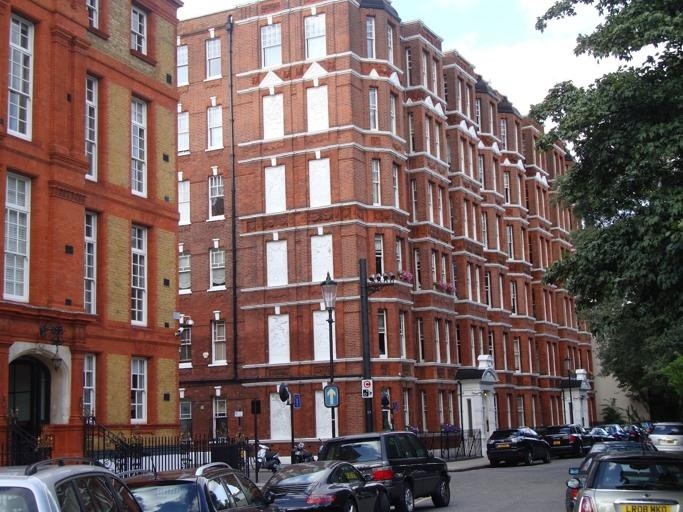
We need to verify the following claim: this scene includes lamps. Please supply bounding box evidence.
[39,324,64,372]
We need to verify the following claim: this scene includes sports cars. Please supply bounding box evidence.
[261,459,392,512]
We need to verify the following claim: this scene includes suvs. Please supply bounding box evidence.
[113,459,274,511]
[315,429,451,512]
[0,455,144,512]
[485,425,553,467]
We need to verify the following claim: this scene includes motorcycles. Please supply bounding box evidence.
[253,438,283,473]
[289,440,315,464]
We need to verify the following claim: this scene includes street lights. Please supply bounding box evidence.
[320,271,339,438]
[564,356,576,424]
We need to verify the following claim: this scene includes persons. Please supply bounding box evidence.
[294,440,312,464]
[265,443,281,474]
[291,441,298,464]
[628,425,638,438]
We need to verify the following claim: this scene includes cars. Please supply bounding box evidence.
[541,419,682,512]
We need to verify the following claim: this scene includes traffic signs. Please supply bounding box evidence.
[293,394,301,408]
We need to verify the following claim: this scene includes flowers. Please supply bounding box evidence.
[399,270,456,294]
